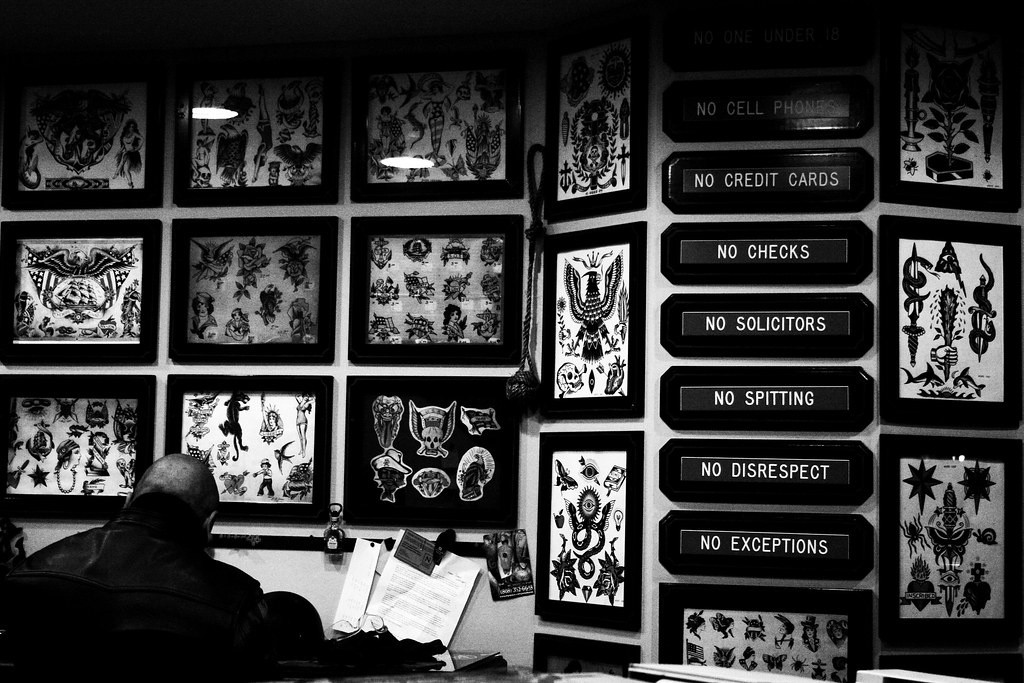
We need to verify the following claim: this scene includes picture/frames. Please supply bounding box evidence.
[0,219,163,367]
[659,583,874,683]
[165,375,334,522]
[349,214,525,365]
[2,66,168,210]
[879,10,1021,213]
[352,47,524,203]
[170,216,338,362]
[535,431,644,632]
[343,376,519,530]
[533,633,640,679]
[0,375,156,518]
[879,433,1024,650]
[175,59,345,206]
[540,222,647,418]
[877,216,1021,430]
[544,14,648,221]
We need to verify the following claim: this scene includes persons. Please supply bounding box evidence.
[0,455,275,683]
